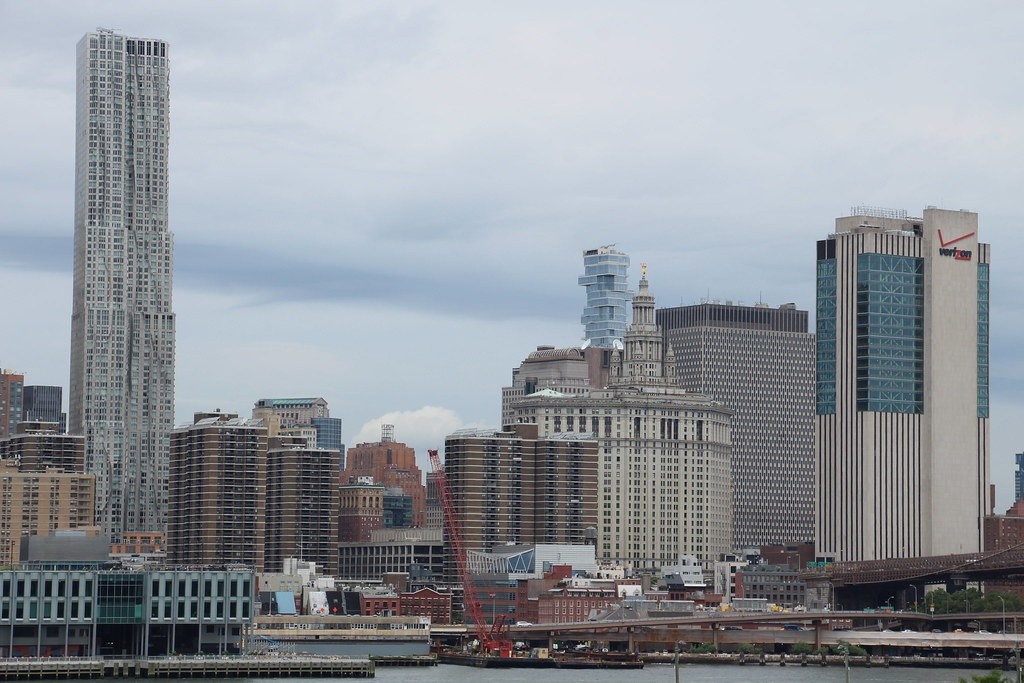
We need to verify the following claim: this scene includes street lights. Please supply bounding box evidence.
[998,596,1005,635]
[926,589,933,617]
[888,596,894,609]
[829,582,834,612]
[910,584,917,612]
[961,589,968,613]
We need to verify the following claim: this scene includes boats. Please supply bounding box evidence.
[549,644,645,669]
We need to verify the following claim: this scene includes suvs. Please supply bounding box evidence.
[516,621,532,628]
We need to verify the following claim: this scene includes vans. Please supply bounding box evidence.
[784,625,805,631]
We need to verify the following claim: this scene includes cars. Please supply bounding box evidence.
[976,629,991,635]
[883,629,894,632]
[953,629,965,634]
[932,628,941,633]
[720,626,741,630]
[902,629,916,633]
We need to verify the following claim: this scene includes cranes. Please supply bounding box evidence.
[427,449,511,656]
